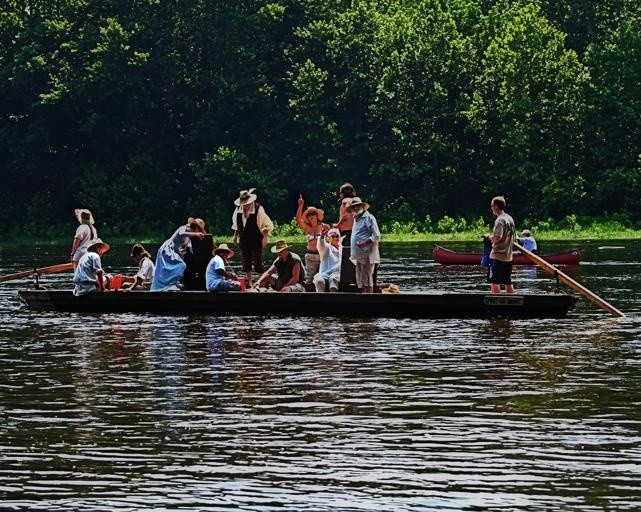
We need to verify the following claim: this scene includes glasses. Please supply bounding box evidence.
[307,214,315,216]
[330,234,339,238]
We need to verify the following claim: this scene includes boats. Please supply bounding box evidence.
[8,284,583,320]
[431,240,584,265]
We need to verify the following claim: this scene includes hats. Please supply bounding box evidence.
[521,229,530,234]
[87,238,110,254]
[75,208,95,225]
[302,206,324,224]
[187,217,207,234]
[234,190,257,206]
[381,284,400,294]
[212,243,235,259]
[346,197,370,212]
[271,241,292,253]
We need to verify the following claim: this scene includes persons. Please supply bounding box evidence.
[116,183,380,292]
[73,238,111,297]
[483,196,516,293]
[513,230,537,256]
[71,209,98,273]
